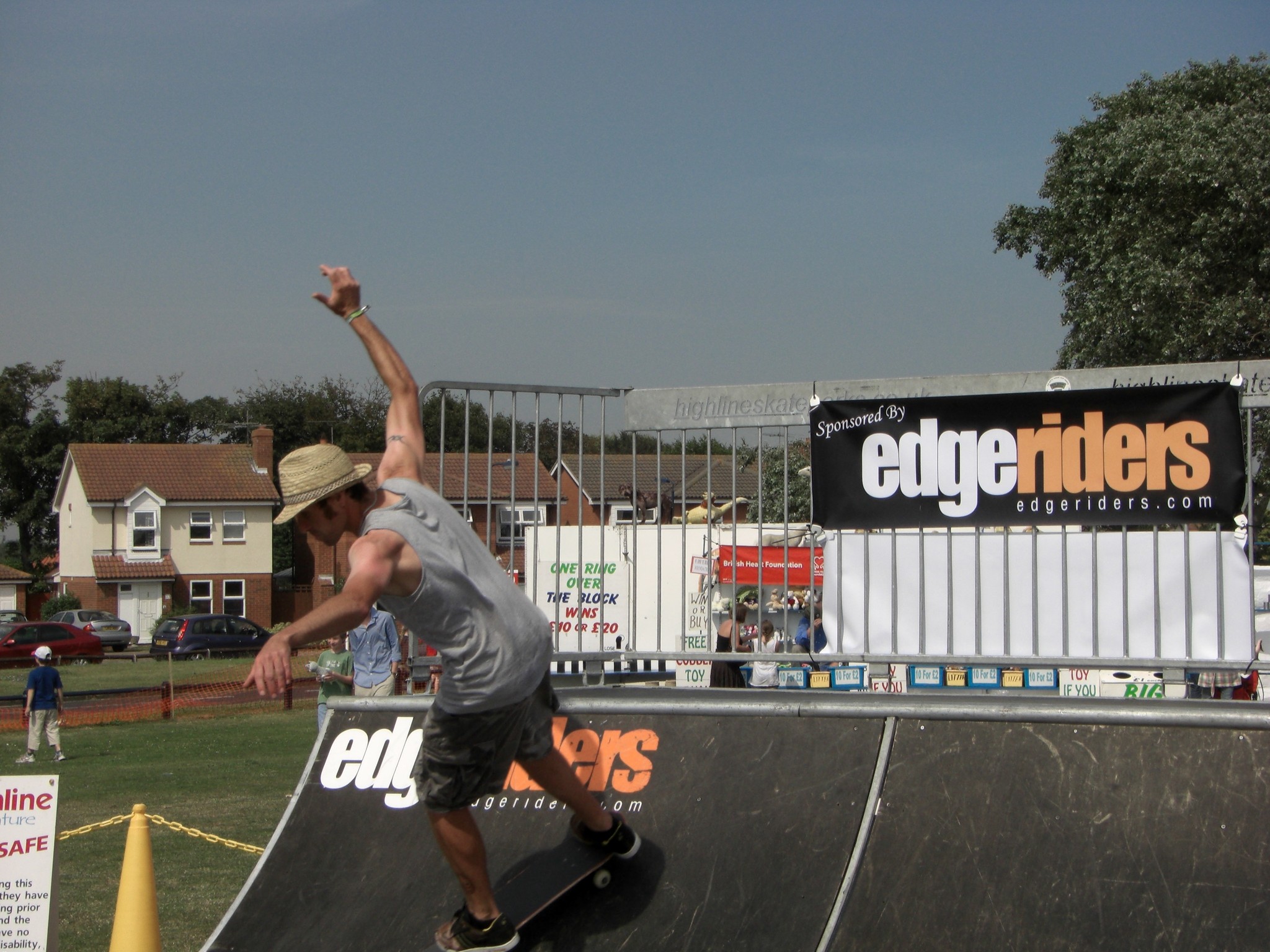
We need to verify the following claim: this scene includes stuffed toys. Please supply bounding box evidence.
[305,661,332,682]
[773,627,790,643]
[739,623,758,641]
[710,585,818,613]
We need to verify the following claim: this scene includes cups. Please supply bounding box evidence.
[1264,602,1269,610]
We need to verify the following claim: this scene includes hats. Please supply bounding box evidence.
[803,606,820,619]
[272,443,372,524]
[31,646,52,660]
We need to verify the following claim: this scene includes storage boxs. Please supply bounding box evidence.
[778,667,810,689]
[809,671,830,688]
[1024,668,1058,688]
[966,666,1004,687]
[827,665,867,687]
[945,669,967,686]
[908,665,944,686]
[1000,670,1024,687]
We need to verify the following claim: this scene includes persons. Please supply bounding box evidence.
[791,595,842,670]
[1187,640,1263,702]
[709,603,758,689]
[15,645,67,763]
[304,606,403,734]
[233,263,644,952]
[746,620,781,689]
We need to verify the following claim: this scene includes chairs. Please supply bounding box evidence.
[214,621,224,633]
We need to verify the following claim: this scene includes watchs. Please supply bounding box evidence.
[391,672,399,678]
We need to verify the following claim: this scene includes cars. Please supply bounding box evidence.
[46,608,132,652]
[0,621,105,668]
[0,609,28,625]
[149,613,298,662]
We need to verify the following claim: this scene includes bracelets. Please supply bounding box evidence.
[344,305,371,324]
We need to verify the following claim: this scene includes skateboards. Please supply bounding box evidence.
[427,812,624,952]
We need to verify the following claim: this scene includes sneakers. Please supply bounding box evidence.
[15,752,36,763]
[434,902,520,952]
[570,811,642,859]
[54,750,66,760]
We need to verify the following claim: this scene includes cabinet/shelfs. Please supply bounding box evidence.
[712,594,816,663]
